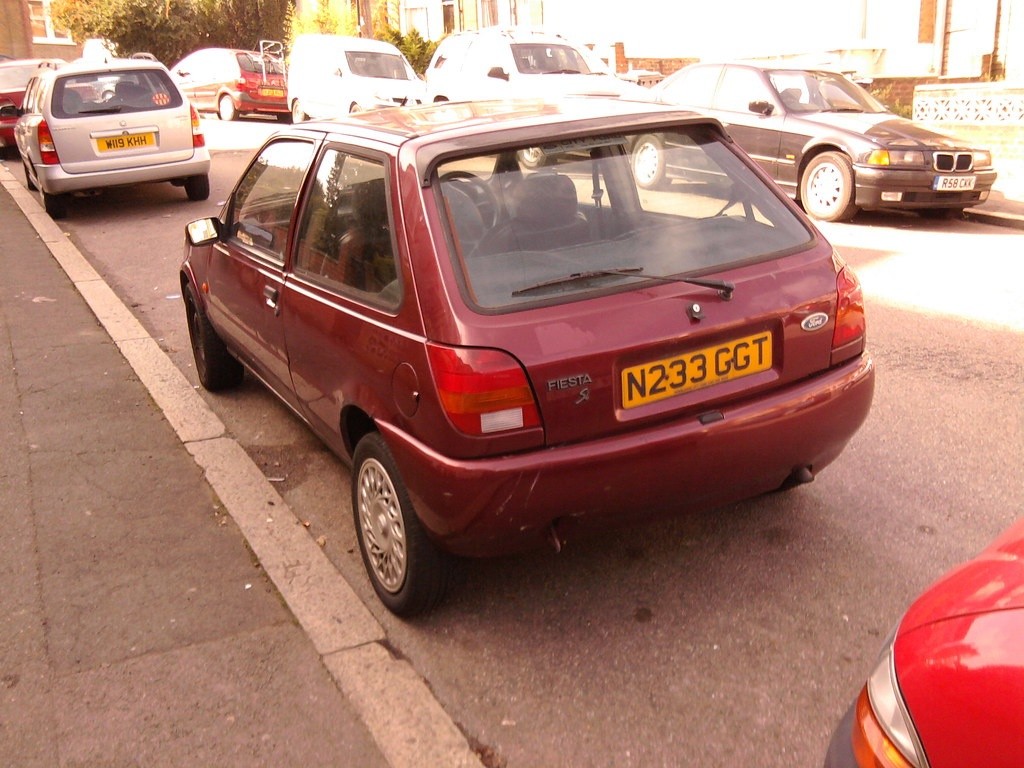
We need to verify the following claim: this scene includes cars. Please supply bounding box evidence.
[414,27,651,105]
[285,34,422,127]
[169,46,295,124]
[641,59,996,223]
[817,507,1024,767]
[1,52,213,219]
[173,97,877,616]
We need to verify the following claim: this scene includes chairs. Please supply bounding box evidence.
[113,81,148,106]
[483,173,589,255]
[63,88,83,115]
[319,176,395,286]
